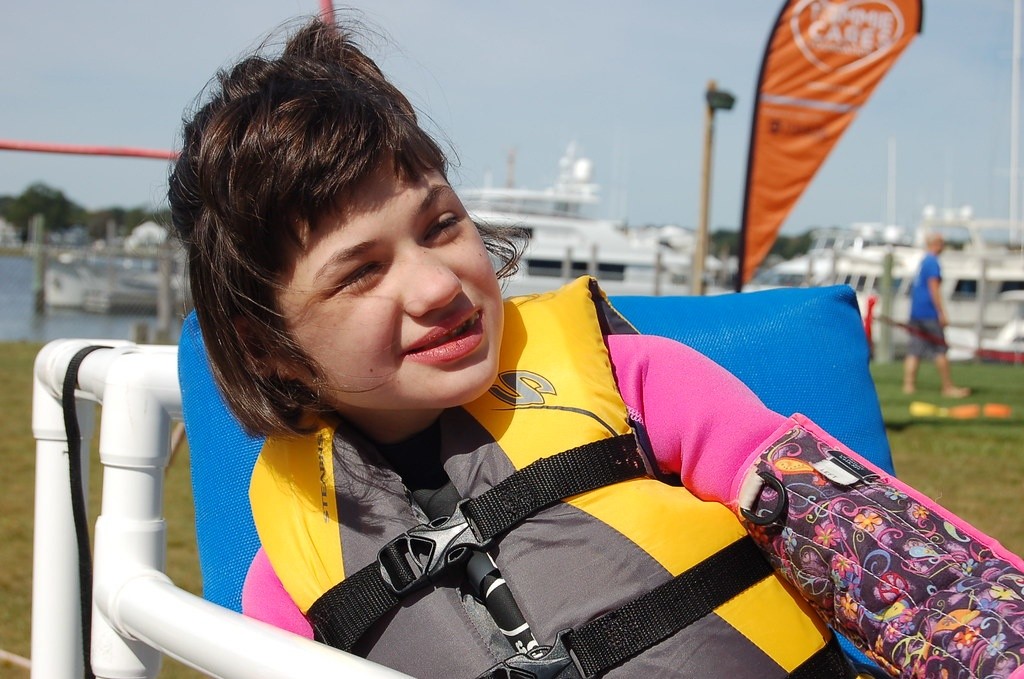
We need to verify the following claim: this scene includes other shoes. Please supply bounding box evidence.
[941,387,972,399]
[903,383,919,397]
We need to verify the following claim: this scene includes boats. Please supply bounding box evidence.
[472,141,1024,363]
[42,222,183,314]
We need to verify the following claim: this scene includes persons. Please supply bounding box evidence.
[166,18,1024,679]
[899,232,972,398]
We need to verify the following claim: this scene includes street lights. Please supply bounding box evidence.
[692,80,738,298]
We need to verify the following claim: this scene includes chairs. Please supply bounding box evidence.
[30,282,899,679]
